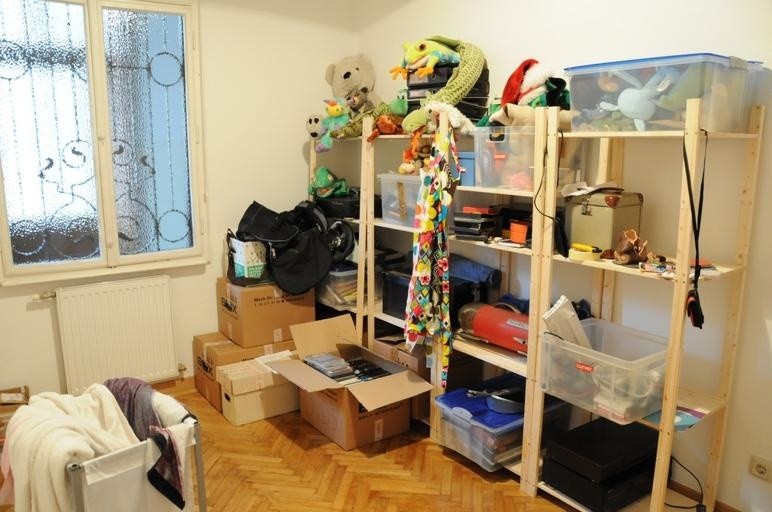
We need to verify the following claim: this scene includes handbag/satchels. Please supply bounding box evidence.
[237,200,357,294]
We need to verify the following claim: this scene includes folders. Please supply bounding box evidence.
[407,65,490,121]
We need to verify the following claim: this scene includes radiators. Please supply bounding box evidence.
[39,274,186,398]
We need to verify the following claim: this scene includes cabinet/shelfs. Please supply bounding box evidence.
[366,107,548,471]
[527,96,768,511]
[308,116,367,343]
[529,102,674,475]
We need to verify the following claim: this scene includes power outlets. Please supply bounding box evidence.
[746,455,772,481]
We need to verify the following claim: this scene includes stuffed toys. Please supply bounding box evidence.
[571,61,711,131]
[305,34,486,199]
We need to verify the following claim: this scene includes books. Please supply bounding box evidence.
[303,344,391,384]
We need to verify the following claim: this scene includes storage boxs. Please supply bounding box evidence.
[0,383,32,447]
[448,152,475,185]
[326,270,384,305]
[563,50,764,132]
[194,334,295,414]
[266,314,434,449]
[215,276,315,346]
[543,318,668,425]
[432,373,572,472]
[374,173,492,234]
[470,125,591,191]
[214,346,298,423]
[370,335,488,421]
